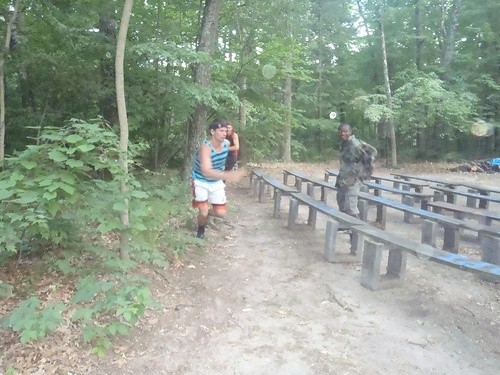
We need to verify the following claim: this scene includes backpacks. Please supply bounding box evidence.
[348,140,378,180]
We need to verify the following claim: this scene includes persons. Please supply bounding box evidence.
[335,123,377,234]
[191,119,240,240]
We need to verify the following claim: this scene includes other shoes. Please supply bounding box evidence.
[197,225,205,239]
[337,228,352,234]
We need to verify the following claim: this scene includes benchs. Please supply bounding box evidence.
[249,168,500,294]
